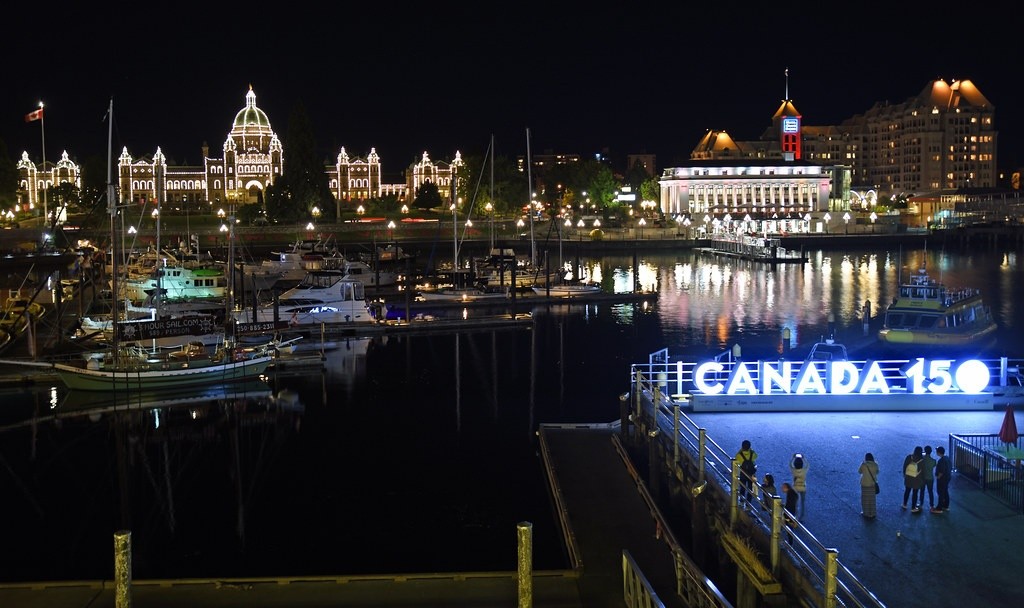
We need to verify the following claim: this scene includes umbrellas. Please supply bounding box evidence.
[998,401,1018,452]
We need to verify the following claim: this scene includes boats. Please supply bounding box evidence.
[880,237,998,346]
[693,225,810,266]
[0,87,666,434]
[927,200,1024,248]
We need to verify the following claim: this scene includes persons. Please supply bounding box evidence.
[900,446,923,512]
[858,453,880,518]
[789,453,810,522]
[930,446,953,513]
[916,445,937,510]
[734,440,758,511]
[781,483,800,548]
[751,473,777,519]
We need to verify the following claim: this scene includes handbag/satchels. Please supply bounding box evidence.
[876,481,880,494]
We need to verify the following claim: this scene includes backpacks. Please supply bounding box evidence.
[739,451,755,481]
[904,456,923,477]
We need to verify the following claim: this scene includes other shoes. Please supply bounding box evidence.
[942,507,950,511]
[930,508,943,513]
[910,508,920,513]
[746,506,751,511]
[739,501,744,506]
[899,504,907,510]
[919,505,924,509]
[930,505,935,509]
